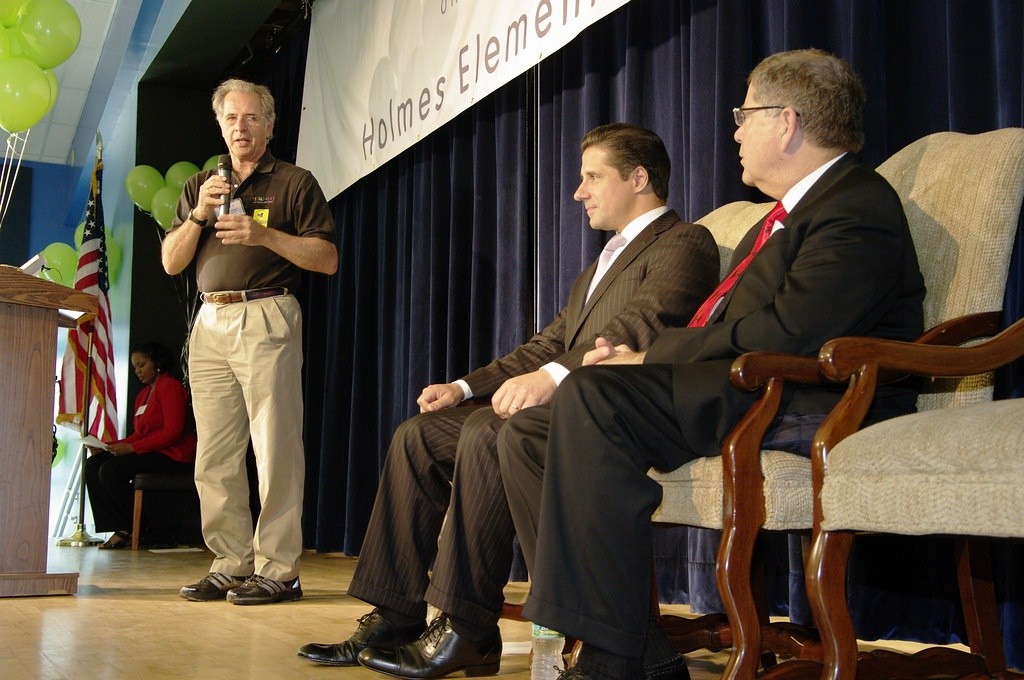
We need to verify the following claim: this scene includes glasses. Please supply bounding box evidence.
[733,105,800,127]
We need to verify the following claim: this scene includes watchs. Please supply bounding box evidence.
[188,208,208,226]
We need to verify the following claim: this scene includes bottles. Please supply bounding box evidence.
[531,622,566,680]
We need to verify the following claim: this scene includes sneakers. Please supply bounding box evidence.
[226,574,303,605]
[179,571,254,602]
[553,652,691,680]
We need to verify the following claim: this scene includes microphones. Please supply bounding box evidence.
[218,155,232,215]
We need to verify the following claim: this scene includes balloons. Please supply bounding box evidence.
[0,0,81,141]
[36,219,124,287]
[126,155,222,232]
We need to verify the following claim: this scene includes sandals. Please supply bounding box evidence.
[99,530,132,549]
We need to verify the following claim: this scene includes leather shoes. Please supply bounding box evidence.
[357,612,502,680]
[296,608,428,667]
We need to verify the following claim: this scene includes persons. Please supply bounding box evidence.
[296,123,720,680]
[84,341,198,550]
[495,50,926,680]
[162,80,338,605]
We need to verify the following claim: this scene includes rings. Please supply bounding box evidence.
[511,405,521,409]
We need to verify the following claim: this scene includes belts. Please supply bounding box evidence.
[204,287,292,305]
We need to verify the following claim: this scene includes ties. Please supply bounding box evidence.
[686,200,787,328]
[585,234,627,305]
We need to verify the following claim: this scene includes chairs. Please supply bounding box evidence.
[502,126,1024,679]
[132,471,196,551]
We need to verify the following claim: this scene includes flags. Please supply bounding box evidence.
[55,156,118,454]
